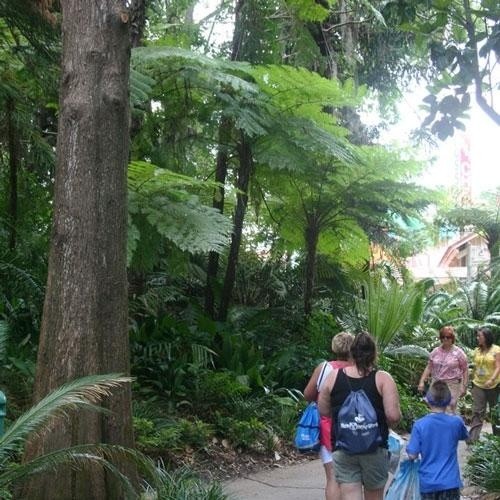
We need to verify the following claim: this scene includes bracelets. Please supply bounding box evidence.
[490,377,494,381]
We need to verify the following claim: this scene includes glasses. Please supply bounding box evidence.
[442,335,451,340]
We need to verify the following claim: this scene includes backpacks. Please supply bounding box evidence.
[334,368,380,456]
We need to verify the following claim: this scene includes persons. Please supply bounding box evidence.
[303,330,356,500]
[402,379,471,500]
[464,327,500,446]
[417,326,469,418]
[317,331,403,500]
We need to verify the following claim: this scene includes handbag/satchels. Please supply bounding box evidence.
[385,458,421,500]
[293,363,326,455]
